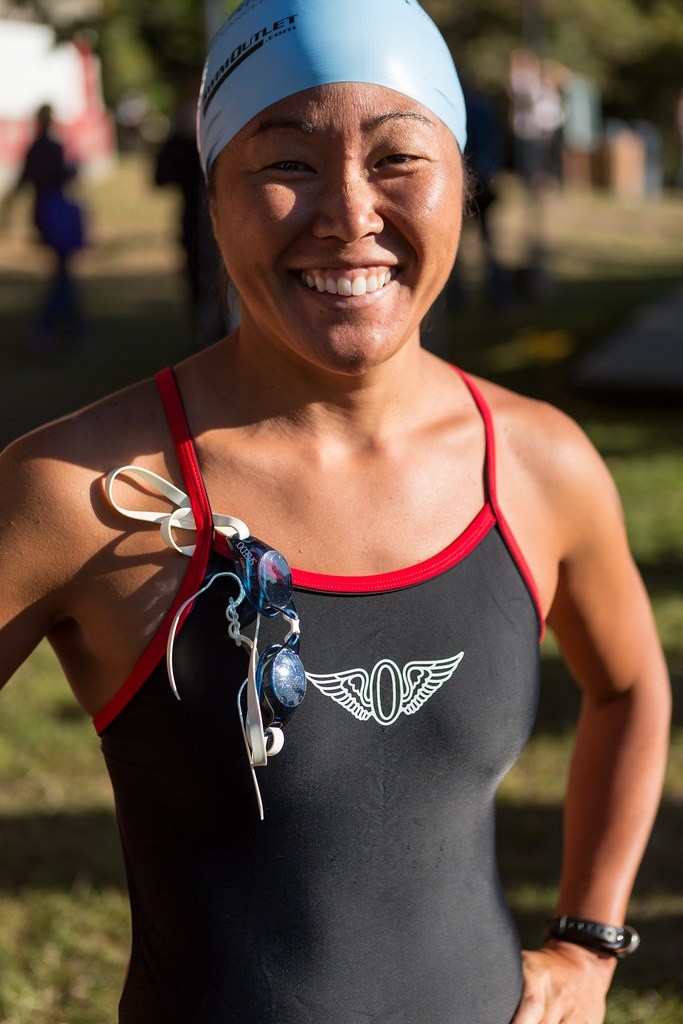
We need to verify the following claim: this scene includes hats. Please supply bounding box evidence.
[196,0,467,183]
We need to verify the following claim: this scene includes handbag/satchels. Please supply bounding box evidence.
[41,186,84,254]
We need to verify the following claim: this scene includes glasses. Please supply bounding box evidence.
[106,466,306,821]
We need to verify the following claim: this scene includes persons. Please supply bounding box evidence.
[35,167,92,350]
[143,50,598,359]
[5,101,85,247]
[4,0,673,1024]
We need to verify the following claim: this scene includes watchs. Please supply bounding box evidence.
[550,912,640,962]
